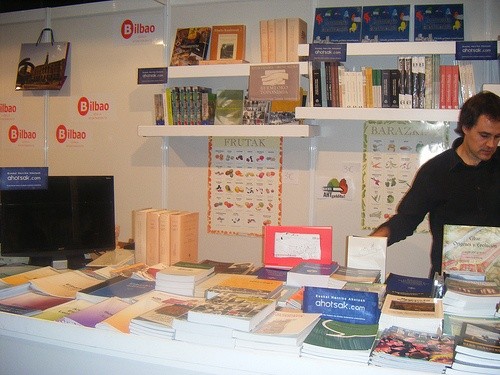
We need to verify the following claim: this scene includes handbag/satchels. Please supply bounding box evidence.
[15,28,71,90]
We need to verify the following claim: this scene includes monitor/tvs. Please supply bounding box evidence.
[0,176,116,269]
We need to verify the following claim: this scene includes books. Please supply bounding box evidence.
[0,208,500,375]
[155,17,307,124]
[312,3,500,109]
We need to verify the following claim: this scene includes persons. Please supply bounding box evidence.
[370,91,500,282]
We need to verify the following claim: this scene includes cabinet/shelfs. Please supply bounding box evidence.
[295,42,499,121]
[138,62,319,137]
